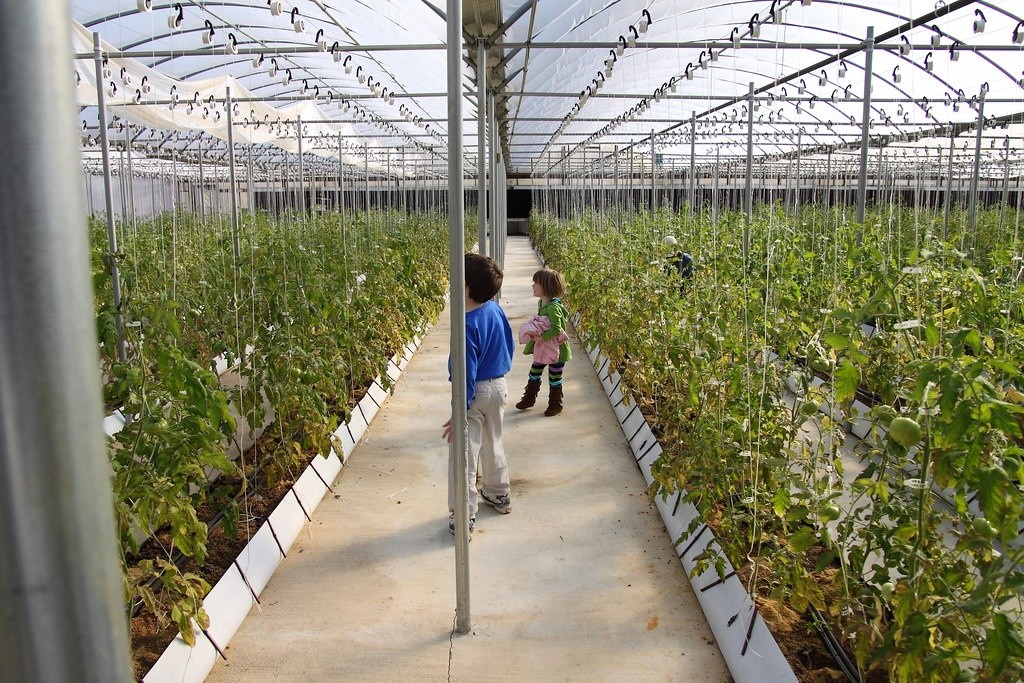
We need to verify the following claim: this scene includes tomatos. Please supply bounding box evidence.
[692,332,922,447]
[110,361,317,435]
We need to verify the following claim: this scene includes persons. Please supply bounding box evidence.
[663,236,694,301]
[516,269,572,416]
[442,253,515,541]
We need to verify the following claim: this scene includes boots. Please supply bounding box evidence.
[544,385,564,416]
[516,380,542,409]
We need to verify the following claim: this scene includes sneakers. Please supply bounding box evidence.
[479,487,512,514]
[449,516,474,542]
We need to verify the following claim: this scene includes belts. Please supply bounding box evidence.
[492,375,504,379]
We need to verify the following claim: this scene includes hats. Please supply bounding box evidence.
[661,236,677,258]
